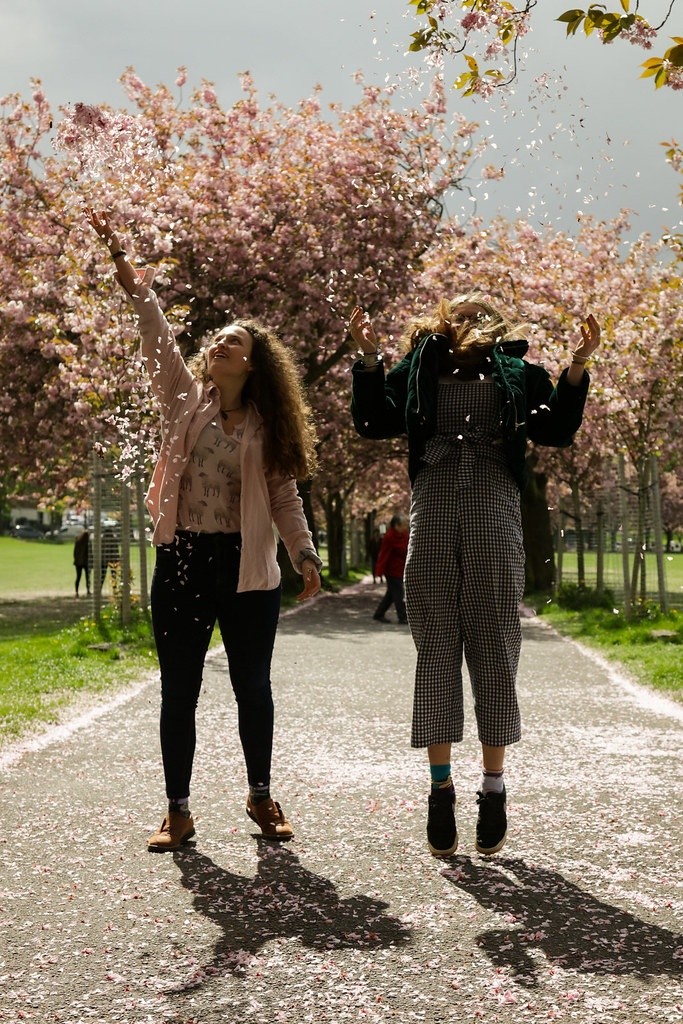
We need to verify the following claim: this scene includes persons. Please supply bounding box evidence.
[347,294,601,856]
[74,531,93,598]
[366,515,410,625]
[82,207,323,849]
[100,529,121,595]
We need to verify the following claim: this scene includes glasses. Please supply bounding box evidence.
[454,312,485,324]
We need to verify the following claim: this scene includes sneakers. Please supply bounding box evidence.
[475,783,508,855]
[426,780,458,856]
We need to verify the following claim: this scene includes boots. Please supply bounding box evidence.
[395,602,408,624]
[372,599,393,624]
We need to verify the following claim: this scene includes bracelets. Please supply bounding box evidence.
[364,352,377,354]
[571,352,590,361]
[112,250,126,259]
[572,360,585,365]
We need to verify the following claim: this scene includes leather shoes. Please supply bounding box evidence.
[148,810,196,851]
[245,795,294,840]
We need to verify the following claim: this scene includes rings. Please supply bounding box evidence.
[597,335,600,337]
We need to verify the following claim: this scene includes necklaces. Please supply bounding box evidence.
[220,406,242,421]
